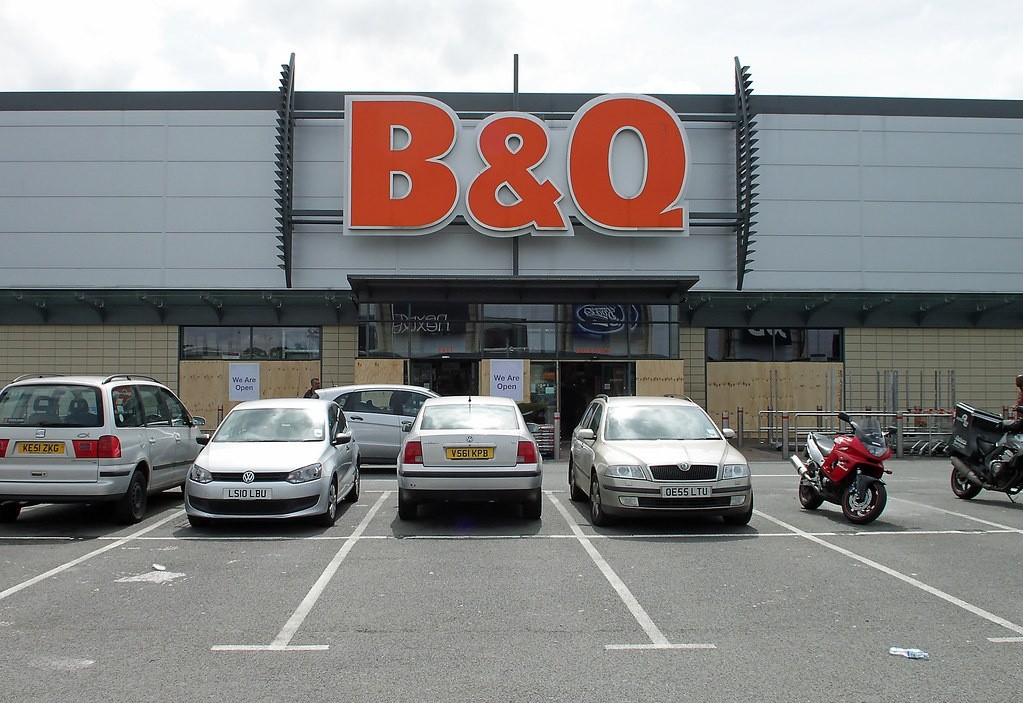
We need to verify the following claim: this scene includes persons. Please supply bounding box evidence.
[1012,375,1023,418]
[303,378,322,399]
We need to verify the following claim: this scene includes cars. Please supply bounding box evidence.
[395,395,544,521]
[304,382,444,466]
[183,395,362,529]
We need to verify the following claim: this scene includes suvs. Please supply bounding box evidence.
[567,393,755,528]
[0,371,211,525]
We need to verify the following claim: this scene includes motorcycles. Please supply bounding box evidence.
[788,411,892,525]
[943,401,1023,505]
